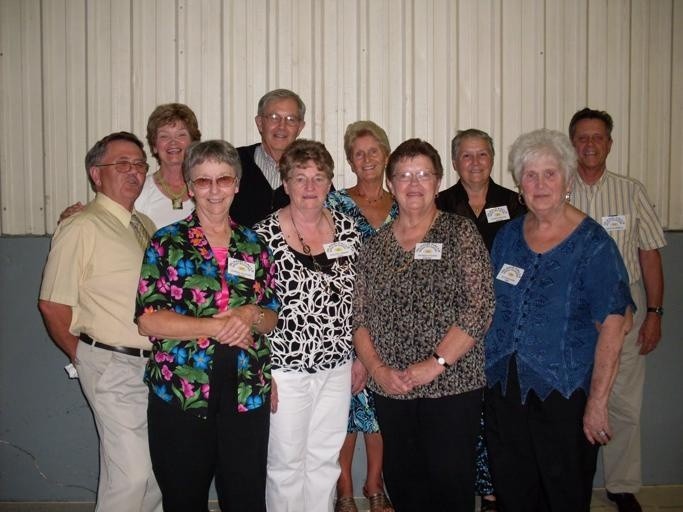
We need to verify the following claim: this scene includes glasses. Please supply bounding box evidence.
[96,161,148,173]
[393,171,436,181]
[191,176,237,185]
[261,113,300,126]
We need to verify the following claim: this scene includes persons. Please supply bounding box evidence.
[35,86,666,512]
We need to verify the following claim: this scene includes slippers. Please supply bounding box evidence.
[336,497,357,512]
[362,488,394,512]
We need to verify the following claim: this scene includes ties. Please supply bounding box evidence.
[130,214,150,250]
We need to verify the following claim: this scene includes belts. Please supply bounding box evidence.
[79,332,151,357]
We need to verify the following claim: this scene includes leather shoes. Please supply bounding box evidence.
[607,490,641,512]
